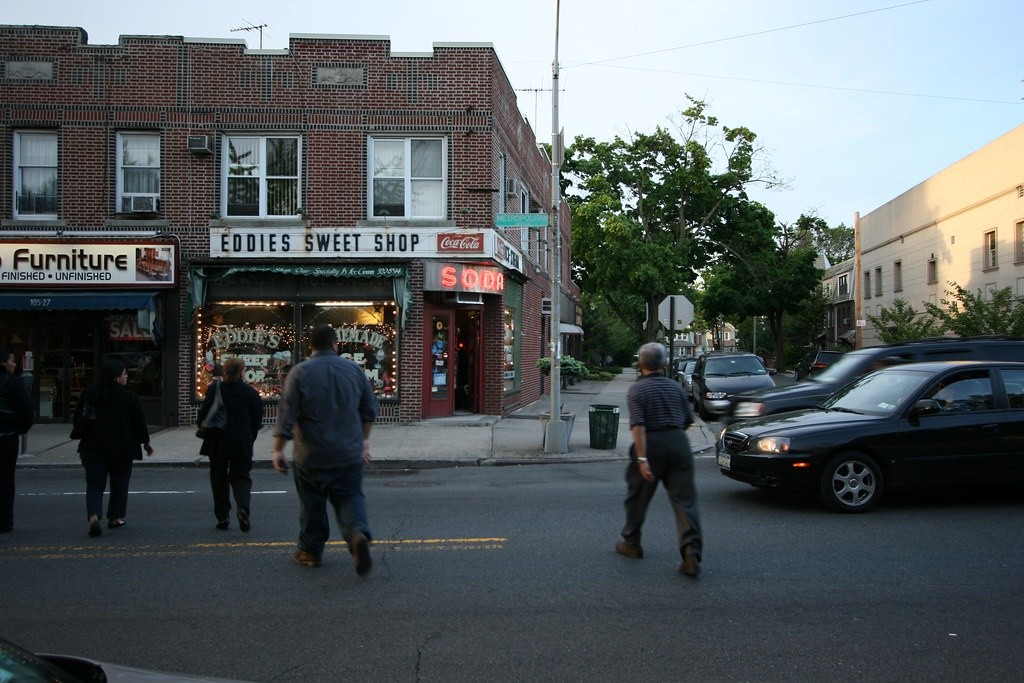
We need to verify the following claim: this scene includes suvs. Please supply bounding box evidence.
[725,334,1024,427]
[691,350,777,422]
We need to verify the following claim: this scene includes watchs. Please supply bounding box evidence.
[637,456,648,464]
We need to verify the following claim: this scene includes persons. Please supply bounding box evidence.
[69,359,154,539]
[614,342,703,576]
[0,346,36,533]
[273,325,376,576]
[195,359,264,532]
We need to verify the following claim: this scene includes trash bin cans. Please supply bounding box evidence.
[588,405,621,450]
[767,356,777,368]
[539,411,575,444]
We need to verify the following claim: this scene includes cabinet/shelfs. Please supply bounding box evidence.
[102,351,165,427]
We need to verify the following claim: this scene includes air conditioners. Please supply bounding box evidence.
[530,208,547,214]
[187,134,211,154]
[507,178,521,199]
[130,195,161,214]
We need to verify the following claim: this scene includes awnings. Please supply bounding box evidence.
[0,286,164,313]
[189,263,412,330]
[560,322,584,335]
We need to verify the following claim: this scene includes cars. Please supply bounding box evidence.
[716,361,1024,513]
[677,361,697,403]
[673,357,698,381]
[795,350,844,381]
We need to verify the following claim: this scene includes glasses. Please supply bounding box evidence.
[119,371,129,375]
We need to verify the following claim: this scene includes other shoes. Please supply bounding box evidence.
[0,526,14,534]
[215,518,230,531]
[88,520,102,538]
[291,549,323,568]
[350,530,371,576]
[106,518,127,529]
[615,538,644,559]
[680,556,702,576]
[236,507,251,534]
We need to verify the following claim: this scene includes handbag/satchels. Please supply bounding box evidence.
[199,379,227,430]
[70,390,96,440]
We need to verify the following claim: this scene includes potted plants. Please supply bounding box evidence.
[537,354,588,391]
